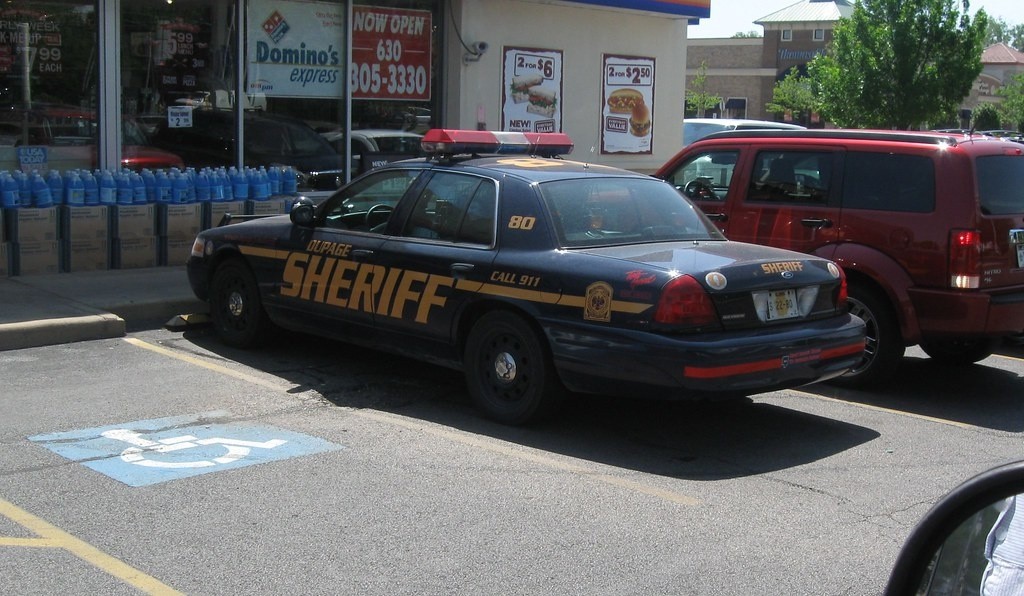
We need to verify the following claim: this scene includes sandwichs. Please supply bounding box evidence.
[510,73,544,103]
[526,88,558,118]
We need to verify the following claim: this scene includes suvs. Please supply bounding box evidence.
[584,119,1023,392]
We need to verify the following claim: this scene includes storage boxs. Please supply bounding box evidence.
[0,199,286,275]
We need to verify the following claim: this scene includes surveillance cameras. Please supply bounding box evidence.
[473,40,490,54]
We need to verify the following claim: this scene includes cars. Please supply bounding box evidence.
[1,102,437,211]
[186,126,869,429]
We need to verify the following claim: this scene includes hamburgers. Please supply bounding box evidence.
[607,89,650,136]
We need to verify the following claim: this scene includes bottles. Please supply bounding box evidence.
[245,166,283,200]
[100,168,171,205]
[283,166,297,195]
[47,168,102,206]
[234,168,248,200]
[78,116,85,137]
[32,170,39,174]
[0,170,32,208]
[85,116,97,137]
[172,166,238,204]
[33,174,53,207]
[168,172,176,181]
[64,114,71,135]
